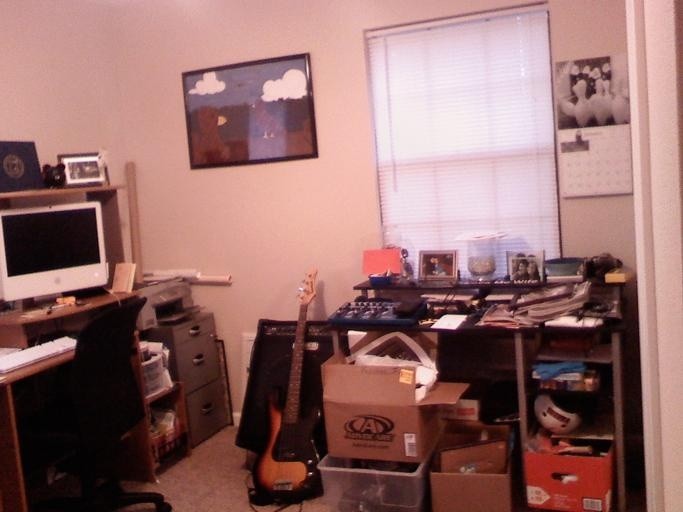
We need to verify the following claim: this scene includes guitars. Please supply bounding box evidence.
[252,267,321,498]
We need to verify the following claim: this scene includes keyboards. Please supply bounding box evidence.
[0,336,77,374]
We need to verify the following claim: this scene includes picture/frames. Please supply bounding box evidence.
[178,52,322,170]
[416,249,457,281]
[55,151,109,188]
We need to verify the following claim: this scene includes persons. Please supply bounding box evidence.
[432,257,445,276]
[423,256,434,273]
[527,263,541,280]
[513,260,527,282]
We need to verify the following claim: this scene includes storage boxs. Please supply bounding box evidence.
[496,335,540,356]
[430,425,512,510]
[320,331,470,463]
[316,453,430,510]
[523,423,616,511]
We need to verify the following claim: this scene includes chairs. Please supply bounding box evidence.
[16,296,174,512]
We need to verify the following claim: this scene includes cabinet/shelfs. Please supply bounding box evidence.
[149,308,224,449]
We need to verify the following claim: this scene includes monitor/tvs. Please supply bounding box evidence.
[0,201,107,312]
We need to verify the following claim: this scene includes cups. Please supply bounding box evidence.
[467,241,497,281]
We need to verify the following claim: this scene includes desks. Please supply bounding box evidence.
[0,187,224,512]
[325,276,632,511]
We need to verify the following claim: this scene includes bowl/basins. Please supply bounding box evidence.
[545,257,583,276]
